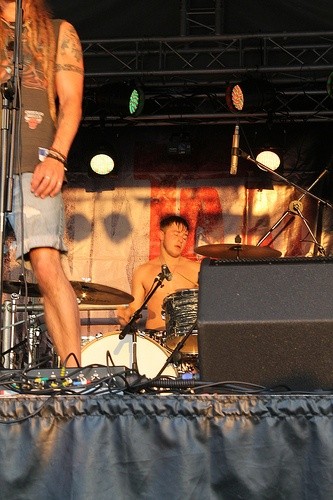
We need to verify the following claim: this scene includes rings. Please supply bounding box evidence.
[45,176,50,179]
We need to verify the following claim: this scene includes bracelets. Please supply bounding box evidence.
[47,147,67,165]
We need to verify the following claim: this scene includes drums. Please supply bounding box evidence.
[80,331,198,380]
[163,288,199,354]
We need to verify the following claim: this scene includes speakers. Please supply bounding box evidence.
[196,257,333,395]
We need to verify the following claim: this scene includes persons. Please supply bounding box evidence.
[0,0,81,367]
[117,215,201,332]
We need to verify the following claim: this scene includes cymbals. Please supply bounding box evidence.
[194,244,282,261]
[68,282,135,305]
[1,279,43,298]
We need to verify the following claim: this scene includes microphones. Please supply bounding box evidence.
[163,265,173,281]
[230,125,239,174]
